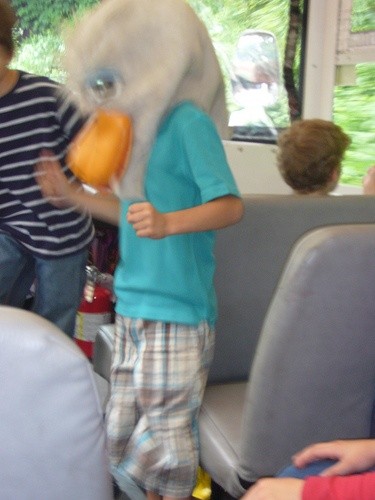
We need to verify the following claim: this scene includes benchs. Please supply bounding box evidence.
[93,195,375,387]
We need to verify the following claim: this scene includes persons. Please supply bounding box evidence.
[238,439,375,500]
[0,0,97,340]
[277,119,352,195]
[34,1,245,499]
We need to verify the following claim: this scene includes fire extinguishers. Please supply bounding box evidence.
[73,263,115,367]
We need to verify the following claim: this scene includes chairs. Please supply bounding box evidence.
[190,224,375,499]
[0,305,114,500]
